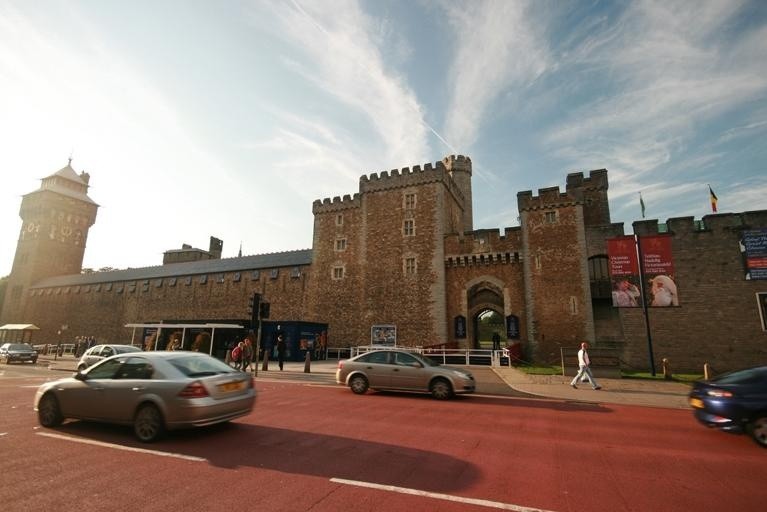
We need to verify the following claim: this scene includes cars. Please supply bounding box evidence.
[32,351,257,442]
[0,343,40,364]
[687,365,767,449]
[75,345,146,373]
[334,348,478,399]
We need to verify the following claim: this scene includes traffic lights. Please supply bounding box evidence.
[246,294,259,320]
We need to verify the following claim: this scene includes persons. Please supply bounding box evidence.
[652,275,679,307]
[313,334,320,360]
[277,335,286,371]
[233,335,255,372]
[385,328,389,335]
[496,332,500,349]
[570,342,602,390]
[74,335,96,358]
[379,328,383,337]
[493,333,496,349]
[320,331,327,360]
[612,276,640,307]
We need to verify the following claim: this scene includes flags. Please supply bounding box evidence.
[640,195,645,218]
[710,187,718,212]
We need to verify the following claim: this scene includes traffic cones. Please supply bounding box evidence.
[224,349,231,365]
[260,349,270,371]
[304,350,310,373]
